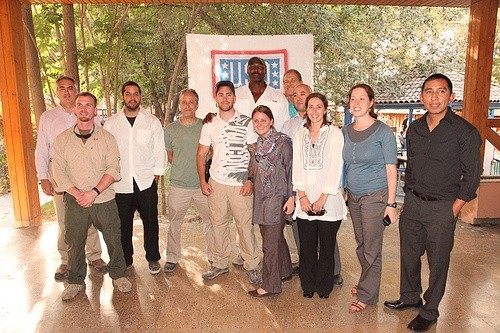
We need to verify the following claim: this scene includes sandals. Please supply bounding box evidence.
[349,299,368,312]
[351,283,358,296]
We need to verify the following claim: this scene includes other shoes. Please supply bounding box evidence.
[248,287,279,297]
[125,264,133,271]
[319,293,328,299]
[303,290,314,298]
[246,269,260,285]
[164,262,178,272]
[201,266,229,279]
[147,260,161,275]
[281,274,292,282]
[333,274,343,287]
[207,259,213,265]
[292,266,299,275]
[232,253,244,266]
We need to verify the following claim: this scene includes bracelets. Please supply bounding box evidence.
[298,195,306,200]
[91,187,100,196]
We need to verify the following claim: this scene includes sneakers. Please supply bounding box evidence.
[61,282,86,300]
[54,264,70,281]
[112,276,132,293]
[89,258,109,273]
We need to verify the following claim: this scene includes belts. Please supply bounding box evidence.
[406,187,441,202]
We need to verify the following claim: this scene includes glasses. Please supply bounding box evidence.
[306,208,328,216]
[73,123,96,139]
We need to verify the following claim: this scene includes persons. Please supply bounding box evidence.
[290,93,348,300]
[383,73,483,333]
[341,83,399,310]
[34,77,108,280]
[50,92,131,299]
[101,80,167,275]
[164,89,214,273]
[195,56,344,285]
[196,81,261,284]
[246,105,296,295]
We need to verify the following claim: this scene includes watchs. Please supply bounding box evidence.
[386,202,398,208]
[247,177,255,183]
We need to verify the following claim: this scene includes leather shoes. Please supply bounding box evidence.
[407,314,437,333]
[384,297,423,311]
